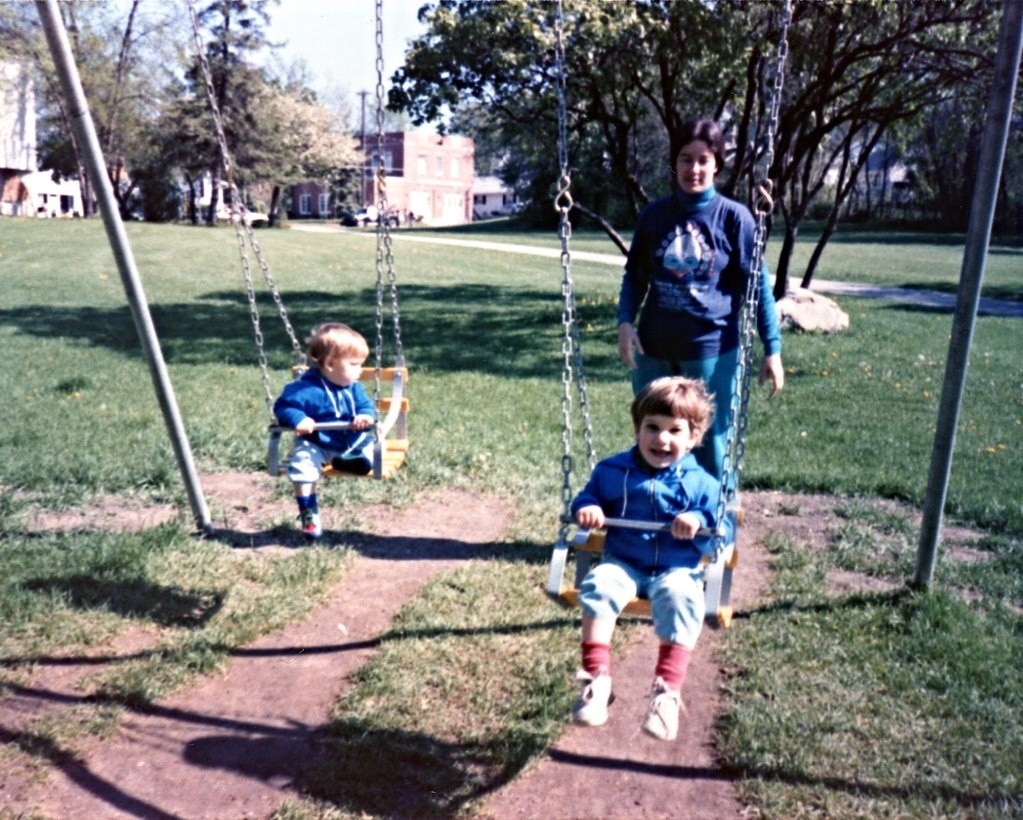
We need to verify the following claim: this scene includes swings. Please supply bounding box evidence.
[540,1,797,630]
[186,2,412,482]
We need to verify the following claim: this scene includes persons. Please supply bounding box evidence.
[570,373,732,744]
[617,118,785,502]
[273,323,378,538]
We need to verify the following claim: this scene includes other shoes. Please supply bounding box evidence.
[332,456,372,476]
[296,509,323,539]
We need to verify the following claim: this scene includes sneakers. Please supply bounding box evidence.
[573,664,612,726]
[642,677,690,742]
